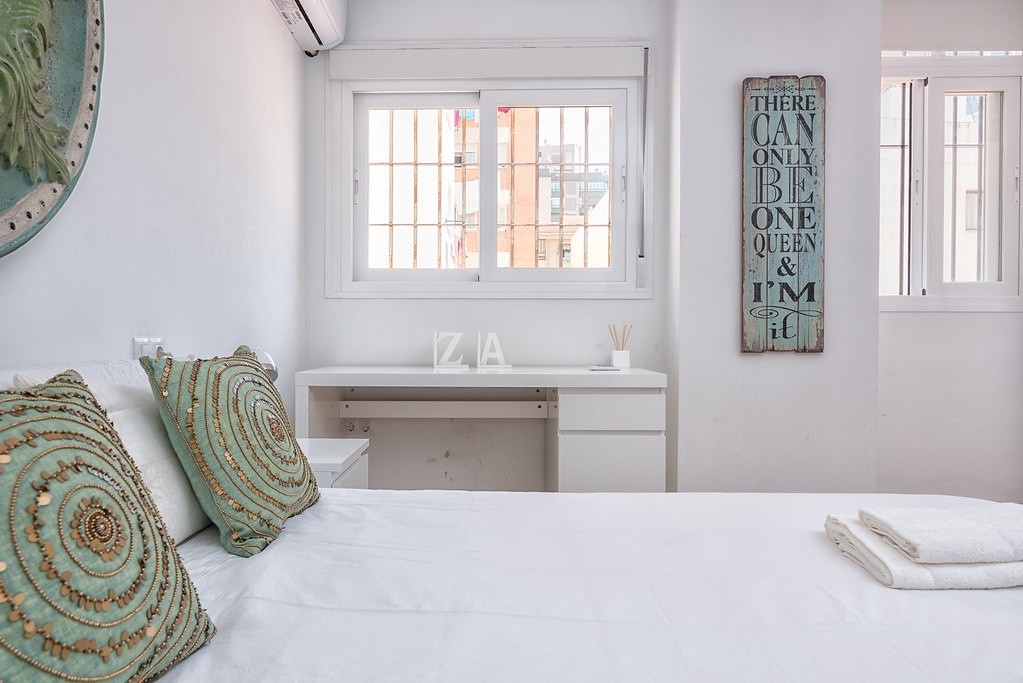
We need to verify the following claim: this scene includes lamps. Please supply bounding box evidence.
[254,348,278,382]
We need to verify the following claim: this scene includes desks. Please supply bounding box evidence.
[293,366,668,494]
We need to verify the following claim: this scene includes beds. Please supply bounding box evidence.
[0,360,1023,682]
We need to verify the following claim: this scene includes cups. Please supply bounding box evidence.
[612,349,631,369]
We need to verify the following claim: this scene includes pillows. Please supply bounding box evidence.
[0,370,218,682]
[14,344,321,559]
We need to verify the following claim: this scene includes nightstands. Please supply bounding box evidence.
[294,437,370,489]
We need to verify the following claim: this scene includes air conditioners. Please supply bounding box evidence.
[270,0,346,52]
